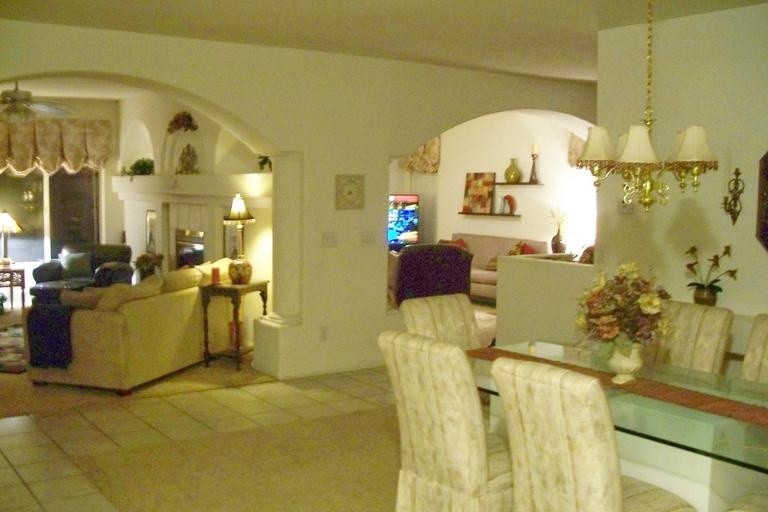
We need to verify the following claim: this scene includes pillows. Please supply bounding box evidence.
[484,240,524,270]
[521,243,536,254]
[58,253,91,278]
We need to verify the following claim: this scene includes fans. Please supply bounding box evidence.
[1,81,73,116]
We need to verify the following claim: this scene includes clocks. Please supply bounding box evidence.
[334,175,365,210]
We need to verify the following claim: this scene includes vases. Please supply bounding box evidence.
[550,224,567,253]
[607,343,643,384]
[504,158,521,182]
[694,288,716,305]
[228,259,253,285]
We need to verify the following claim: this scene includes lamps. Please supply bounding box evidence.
[0,82,36,123]
[223,194,256,259]
[576,0,718,212]
[0,213,21,264]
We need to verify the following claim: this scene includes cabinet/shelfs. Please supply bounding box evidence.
[458,182,539,217]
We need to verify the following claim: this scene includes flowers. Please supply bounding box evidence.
[573,262,672,360]
[685,245,737,297]
[546,209,568,234]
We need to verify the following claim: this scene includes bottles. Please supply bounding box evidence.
[212,267,220,286]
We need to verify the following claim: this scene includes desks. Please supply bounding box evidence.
[464,339,768,512]
[0,263,25,309]
[199,280,270,371]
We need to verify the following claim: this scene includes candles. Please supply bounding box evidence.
[531,142,538,154]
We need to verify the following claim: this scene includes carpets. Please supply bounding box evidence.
[0,324,26,374]
[70,406,400,512]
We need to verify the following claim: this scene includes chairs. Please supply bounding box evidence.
[399,292,482,348]
[378,330,512,512]
[491,356,697,512]
[648,301,734,374]
[740,315,768,384]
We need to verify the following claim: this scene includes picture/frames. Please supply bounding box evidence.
[463,172,495,213]
[224,224,243,257]
[146,209,157,253]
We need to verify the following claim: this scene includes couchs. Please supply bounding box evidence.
[452,234,547,299]
[22,257,230,395]
[33,244,134,307]
[388,244,474,309]
